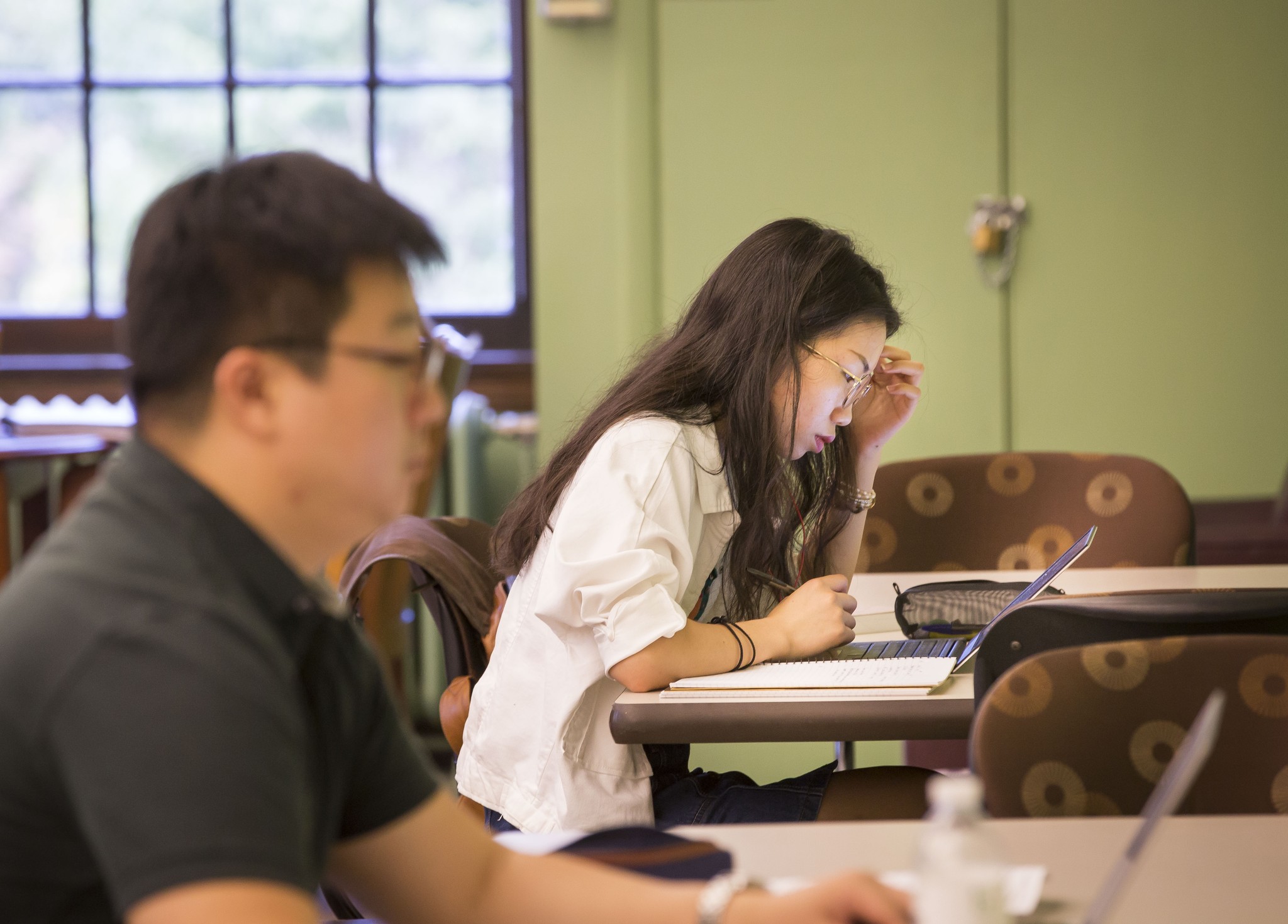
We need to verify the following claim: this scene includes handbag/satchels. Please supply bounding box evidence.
[895,578,1064,639]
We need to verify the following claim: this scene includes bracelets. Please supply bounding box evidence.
[697,872,761,924]
[827,477,876,509]
[708,614,756,672]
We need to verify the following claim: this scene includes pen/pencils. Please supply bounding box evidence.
[920,623,987,639]
[745,566,796,595]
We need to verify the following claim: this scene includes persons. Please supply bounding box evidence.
[453,216,945,832]
[0,151,918,924]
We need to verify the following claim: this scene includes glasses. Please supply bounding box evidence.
[212,338,445,380]
[796,338,873,409]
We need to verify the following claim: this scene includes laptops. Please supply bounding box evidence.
[786,526,1099,676]
[1086,691,1229,924]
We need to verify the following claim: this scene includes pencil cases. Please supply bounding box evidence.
[892,580,1066,640]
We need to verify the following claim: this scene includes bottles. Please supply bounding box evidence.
[909,774,1009,924]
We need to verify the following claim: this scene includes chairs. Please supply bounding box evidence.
[852,450,1194,575]
[337,515,497,775]
[973,587,1288,711]
[967,635,1288,817]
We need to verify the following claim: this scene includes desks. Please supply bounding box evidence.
[492,564,1288,924]
